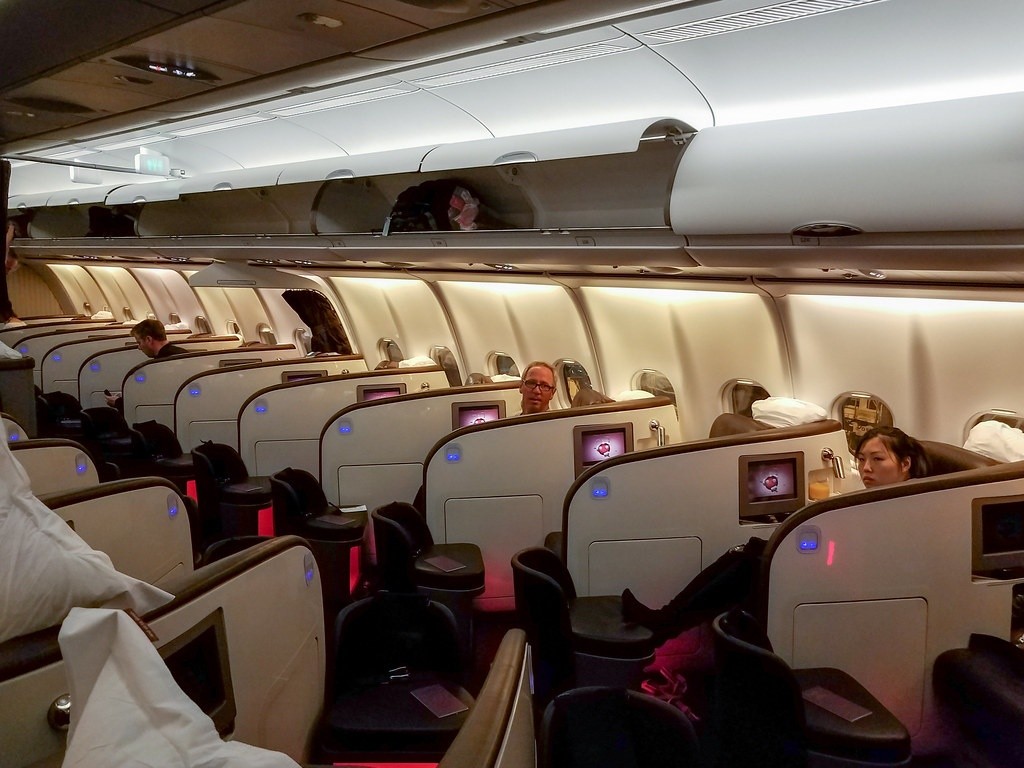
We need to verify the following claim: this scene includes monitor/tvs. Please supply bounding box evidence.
[358,383,406,402]
[739,451,805,518]
[157,608,238,734]
[971,494,1024,579]
[219,359,262,368]
[574,423,634,480]
[452,400,506,432]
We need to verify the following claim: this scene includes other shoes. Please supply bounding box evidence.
[621,588,681,635]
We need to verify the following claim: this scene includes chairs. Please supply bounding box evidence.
[34,390,910,768]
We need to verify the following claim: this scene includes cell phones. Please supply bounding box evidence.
[104,389,112,396]
[282,370,327,384]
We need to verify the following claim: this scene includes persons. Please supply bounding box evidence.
[514,360,558,415]
[854,426,941,489]
[0,297,27,330]
[105,319,191,415]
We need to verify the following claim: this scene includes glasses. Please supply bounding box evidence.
[522,380,555,391]
